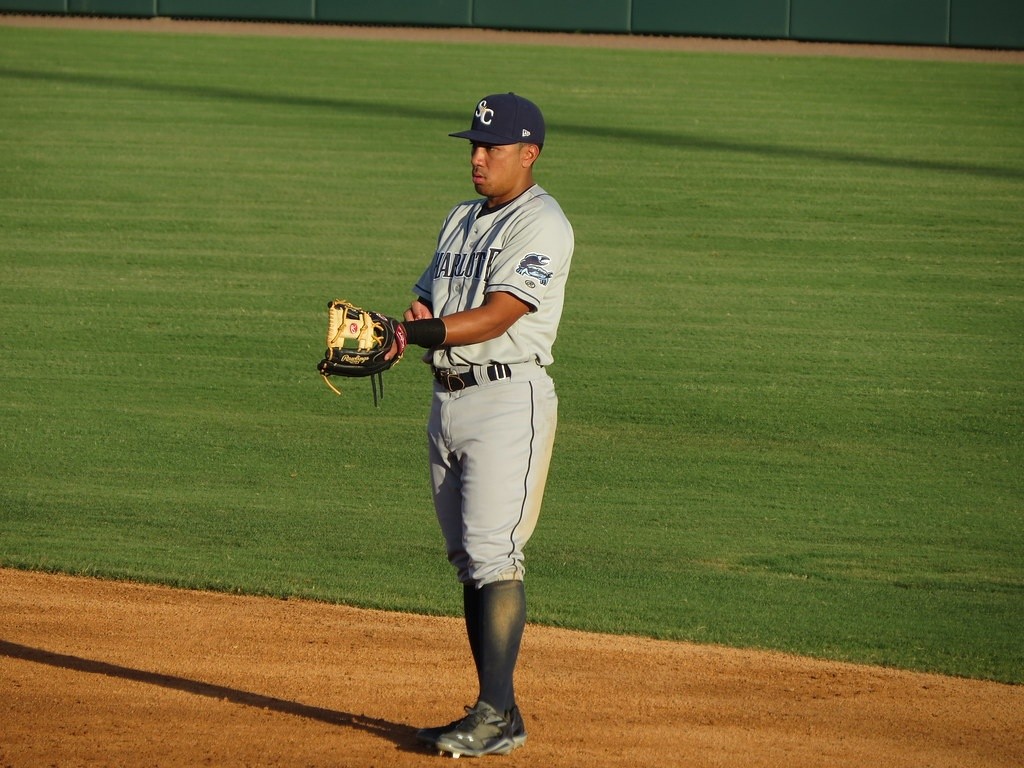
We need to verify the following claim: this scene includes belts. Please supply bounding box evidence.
[435,363,510,392]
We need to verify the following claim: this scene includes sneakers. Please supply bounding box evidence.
[434,701,516,758]
[417,705,526,748]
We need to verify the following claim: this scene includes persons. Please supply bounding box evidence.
[318,93,576,757]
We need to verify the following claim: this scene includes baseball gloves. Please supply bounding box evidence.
[315,297,408,379]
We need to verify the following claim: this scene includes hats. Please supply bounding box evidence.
[449,92,545,146]
